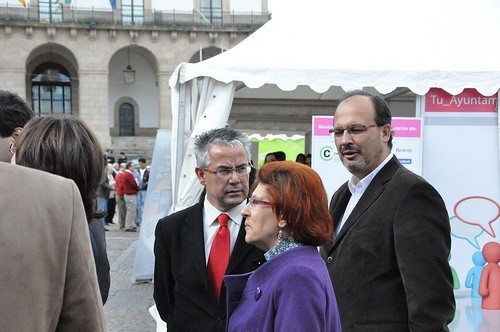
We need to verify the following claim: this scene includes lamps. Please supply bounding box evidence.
[122,46,136,84]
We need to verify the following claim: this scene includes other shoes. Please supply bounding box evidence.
[105,220,141,232]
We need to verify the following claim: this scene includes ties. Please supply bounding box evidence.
[207,213,231,305]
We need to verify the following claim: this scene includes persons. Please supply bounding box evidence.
[263,151,312,168]
[96,149,150,233]
[154,126,270,332]
[0,86,36,162]
[0,161,108,332]
[319,90,456,332]
[223,160,343,332]
[9,116,108,307]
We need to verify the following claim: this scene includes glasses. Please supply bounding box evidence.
[8,142,17,154]
[329,124,380,138]
[247,197,274,208]
[204,163,251,175]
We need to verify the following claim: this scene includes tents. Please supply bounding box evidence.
[169,2,499,215]
